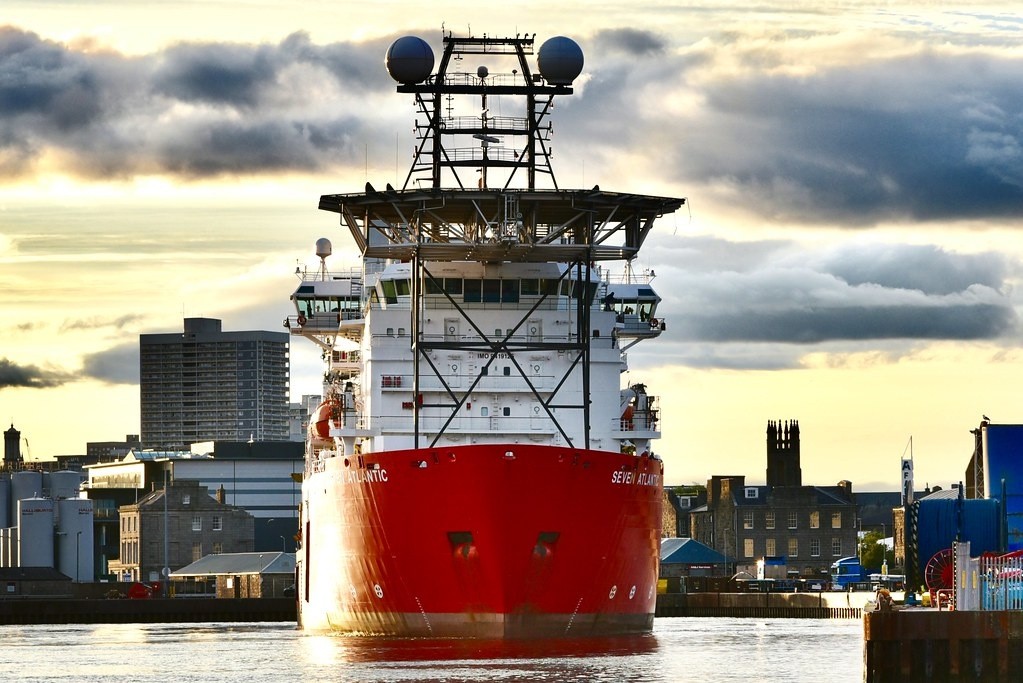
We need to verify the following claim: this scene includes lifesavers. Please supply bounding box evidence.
[649,319,658,328]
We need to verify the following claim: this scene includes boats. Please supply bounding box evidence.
[284,32,685,639]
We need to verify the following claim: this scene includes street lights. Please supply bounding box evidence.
[881,523,885,558]
[77,531,82,581]
[280,536,286,552]
[725,529,728,576]
[165,460,174,598]
[858,518,862,565]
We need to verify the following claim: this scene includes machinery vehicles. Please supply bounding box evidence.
[25,438,31,461]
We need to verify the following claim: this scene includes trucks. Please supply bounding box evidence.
[830,557,906,590]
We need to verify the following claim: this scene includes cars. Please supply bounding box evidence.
[283,584,295,598]
